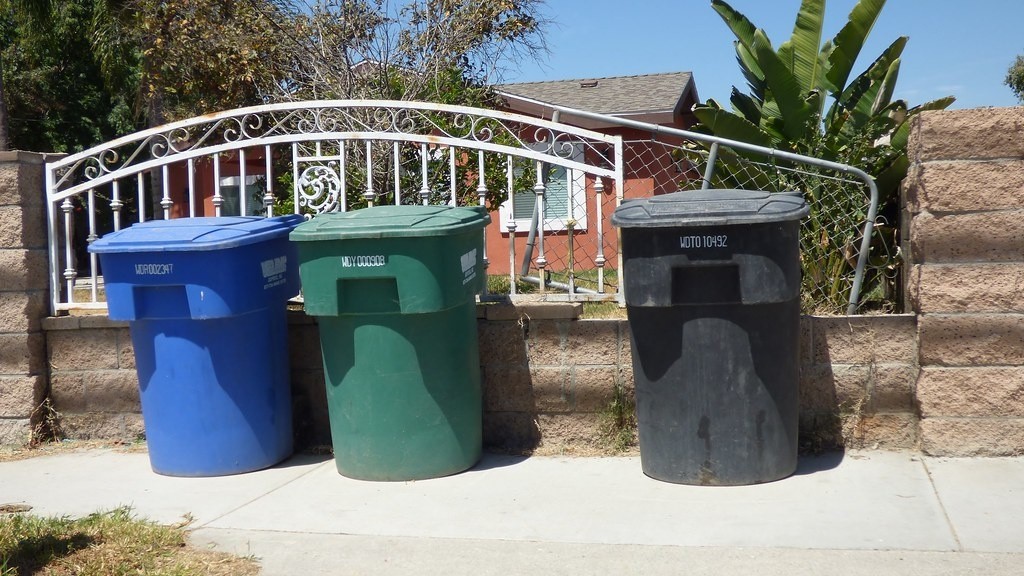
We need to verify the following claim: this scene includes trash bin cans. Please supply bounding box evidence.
[87,214,308,478]
[610,188,811,487]
[290,204,494,482]
